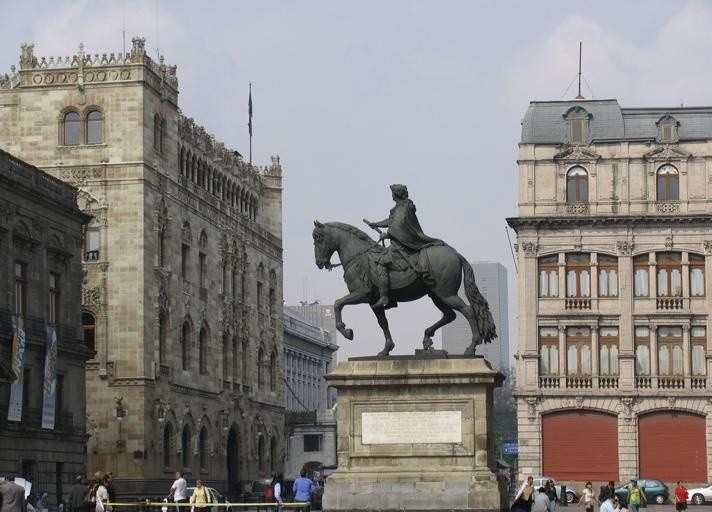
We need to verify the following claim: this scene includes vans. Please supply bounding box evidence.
[247,478,273,503]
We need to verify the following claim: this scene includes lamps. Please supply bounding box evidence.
[113,398,128,422]
[152,400,166,423]
[218,412,231,432]
[252,415,263,437]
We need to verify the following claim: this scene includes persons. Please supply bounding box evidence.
[274,472,286,511]
[530,487,551,512]
[577,480,628,512]
[511,476,534,512]
[675,480,690,511]
[0,473,27,512]
[69,470,118,512]
[189,479,212,512]
[625,479,647,512]
[370,184,447,308]
[291,468,317,512]
[544,479,558,512]
[167,470,188,512]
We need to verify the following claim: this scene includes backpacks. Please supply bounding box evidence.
[264,487,274,504]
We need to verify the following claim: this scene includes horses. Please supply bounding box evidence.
[311,221,499,358]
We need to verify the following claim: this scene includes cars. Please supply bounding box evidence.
[614,478,670,505]
[158,487,233,512]
[523,478,579,503]
[685,483,712,505]
